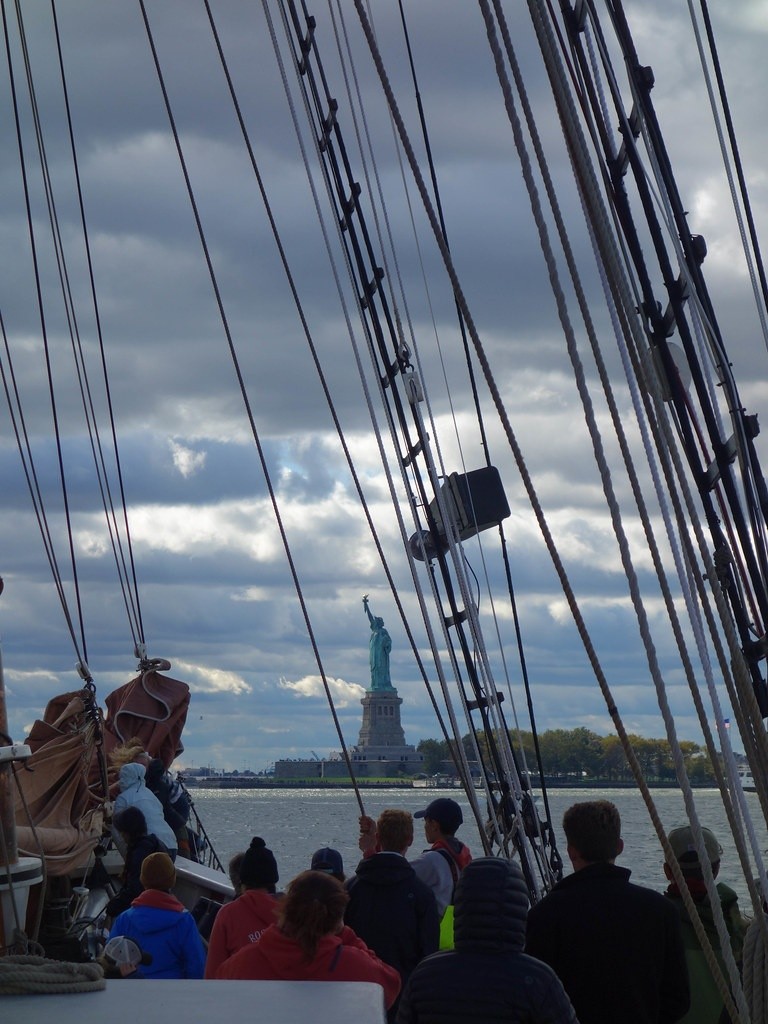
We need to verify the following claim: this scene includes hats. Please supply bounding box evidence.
[311,847,343,874]
[111,806,147,836]
[103,935,152,969]
[413,797,463,833]
[665,825,723,869]
[241,836,278,885]
[140,852,177,891]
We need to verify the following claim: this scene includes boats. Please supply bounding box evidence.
[738,764,757,792]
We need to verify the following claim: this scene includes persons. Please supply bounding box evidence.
[310,849,349,885]
[395,857,581,1024]
[205,838,288,980]
[663,826,756,1024]
[344,810,441,1024]
[87,739,208,865]
[525,801,691,1024]
[105,807,177,917]
[94,852,209,979]
[214,870,401,1013]
[357,798,474,922]
[364,603,397,692]
